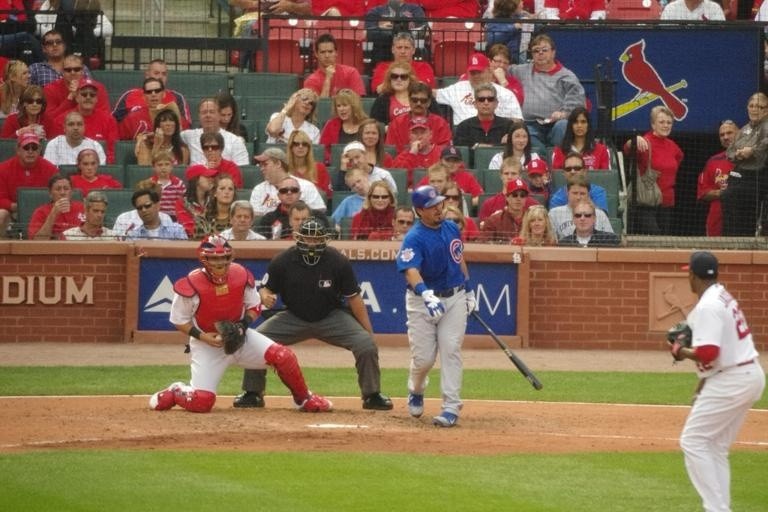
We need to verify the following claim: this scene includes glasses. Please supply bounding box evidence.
[143,88,164,95]
[45,39,63,46]
[202,144,221,152]
[23,144,39,152]
[80,92,97,98]
[492,57,510,66]
[134,203,154,211]
[531,47,552,53]
[63,66,82,73]
[278,73,429,199]
[476,95,497,103]
[506,165,593,218]
[26,97,43,105]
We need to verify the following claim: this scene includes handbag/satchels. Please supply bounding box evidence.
[626,140,664,211]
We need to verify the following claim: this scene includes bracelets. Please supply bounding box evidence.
[465,279,473,293]
[414,282,428,297]
[189,327,202,340]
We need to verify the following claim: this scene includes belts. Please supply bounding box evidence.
[407,282,464,299]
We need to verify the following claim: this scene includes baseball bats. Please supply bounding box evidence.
[471,310,543,390]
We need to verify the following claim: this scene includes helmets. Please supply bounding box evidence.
[410,183,448,210]
[196,232,234,286]
[291,216,331,266]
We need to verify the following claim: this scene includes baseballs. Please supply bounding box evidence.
[215,335,223,341]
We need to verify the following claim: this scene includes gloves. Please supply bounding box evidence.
[463,280,478,315]
[421,288,448,321]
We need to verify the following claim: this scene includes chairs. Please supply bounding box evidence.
[0,0,767,244]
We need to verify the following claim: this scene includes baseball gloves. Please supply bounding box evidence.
[666,323,691,361]
[214,319,248,354]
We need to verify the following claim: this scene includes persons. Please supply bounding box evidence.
[396,185,476,427]
[623,106,685,236]
[220,200,266,240]
[234,218,393,410]
[150,234,333,413]
[667,252,766,512]
[235,1,620,248]
[697,92,768,236]
[659,1,768,75]
[1,0,249,242]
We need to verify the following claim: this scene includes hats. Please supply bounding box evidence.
[440,146,462,162]
[253,146,290,167]
[17,132,41,149]
[684,249,719,281]
[467,52,490,72]
[148,101,182,128]
[78,76,100,91]
[185,163,219,182]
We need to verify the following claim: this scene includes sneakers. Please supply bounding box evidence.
[148,382,185,411]
[407,392,424,418]
[291,390,333,413]
[361,392,393,410]
[232,391,266,408]
[432,411,458,427]
